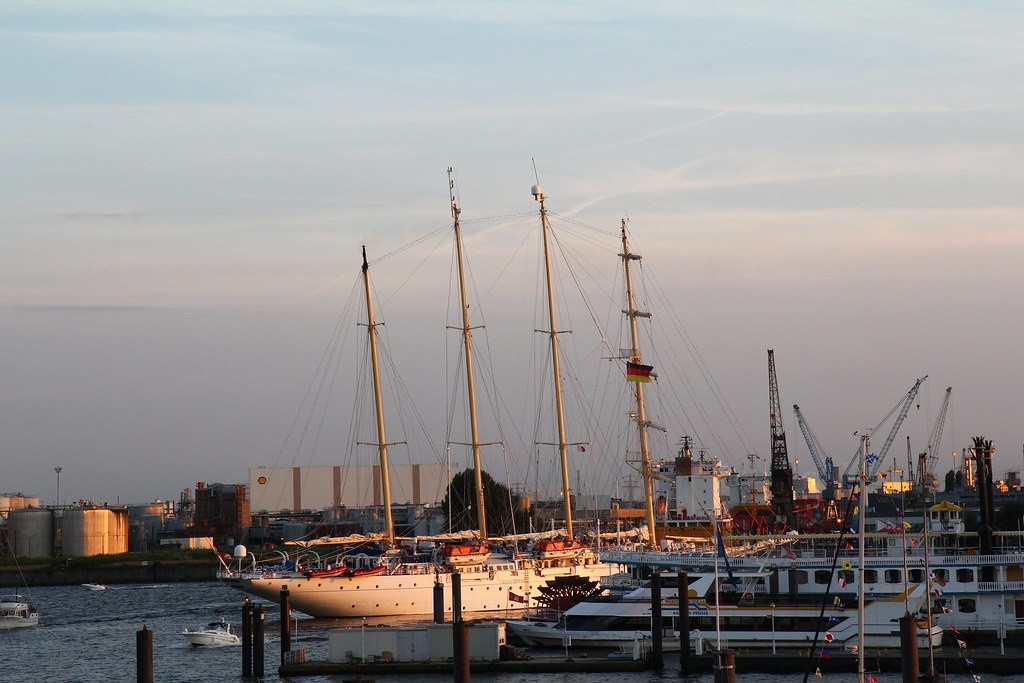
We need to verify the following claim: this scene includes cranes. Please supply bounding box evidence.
[766,347,799,541]
[913,384,952,509]
[837,373,930,497]
[792,404,835,488]
[906,435,916,497]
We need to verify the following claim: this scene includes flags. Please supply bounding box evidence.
[965,658,974,666]
[942,607,953,615]
[824,631,834,642]
[846,543,854,550]
[935,588,945,597]
[929,572,935,578]
[934,570,946,586]
[820,649,830,660]
[627,361,654,384]
[717,526,727,557]
[842,560,852,570]
[816,668,823,678]
[973,675,981,683]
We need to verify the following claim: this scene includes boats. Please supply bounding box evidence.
[0,593,39,631]
[179,617,241,651]
[81,582,116,591]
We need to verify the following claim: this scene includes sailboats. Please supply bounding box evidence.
[208,154,1024,659]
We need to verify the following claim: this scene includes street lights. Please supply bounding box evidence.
[54,464,62,506]
[952,449,957,488]
[795,457,800,486]
